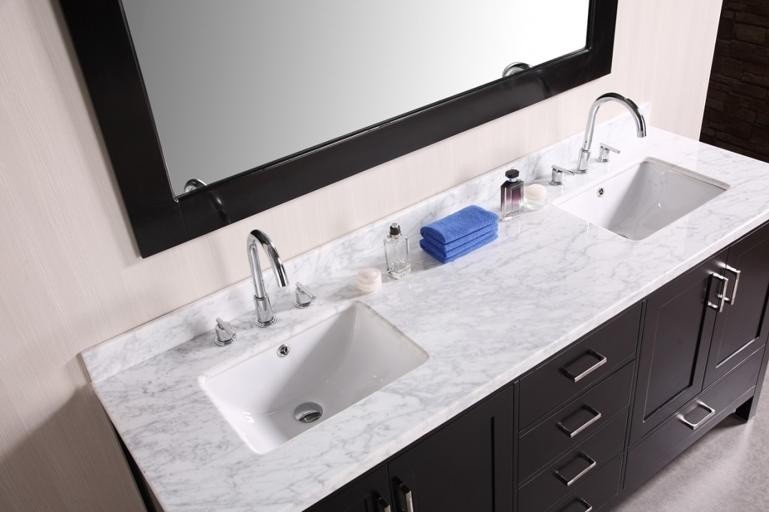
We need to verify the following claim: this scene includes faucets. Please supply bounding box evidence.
[245,228,289,330]
[501,61,552,99]
[574,92,647,175]
[182,177,232,226]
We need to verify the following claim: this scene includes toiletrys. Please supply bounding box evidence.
[500,167,524,222]
[524,183,548,211]
[355,267,384,292]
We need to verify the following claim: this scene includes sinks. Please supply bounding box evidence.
[550,155,731,241]
[197,300,430,457]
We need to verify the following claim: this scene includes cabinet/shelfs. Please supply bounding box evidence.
[303,381,517,510]
[489,298,644,512]
[621,223,768,497]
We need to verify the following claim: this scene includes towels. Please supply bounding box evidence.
[420,205,497,264]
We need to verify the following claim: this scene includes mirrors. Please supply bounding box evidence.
[57,1,618,258]
